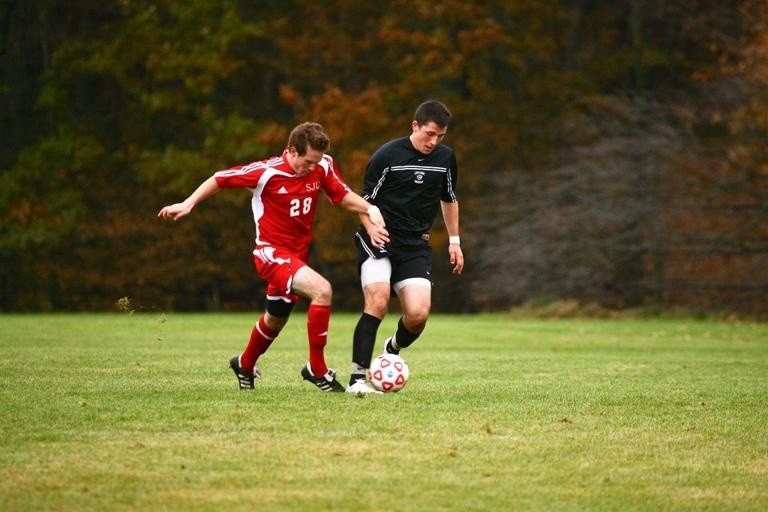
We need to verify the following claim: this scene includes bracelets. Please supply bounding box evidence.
[448,235,461,244]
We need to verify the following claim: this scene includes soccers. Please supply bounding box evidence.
[368,353,409,392]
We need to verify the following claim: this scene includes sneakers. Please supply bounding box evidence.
[301,360,346,392]
[383,337,401,356]
[229,353,261,390]
[345,378,384,394]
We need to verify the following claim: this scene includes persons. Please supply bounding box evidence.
[343,100,465,394]
[156,120,391,394]
